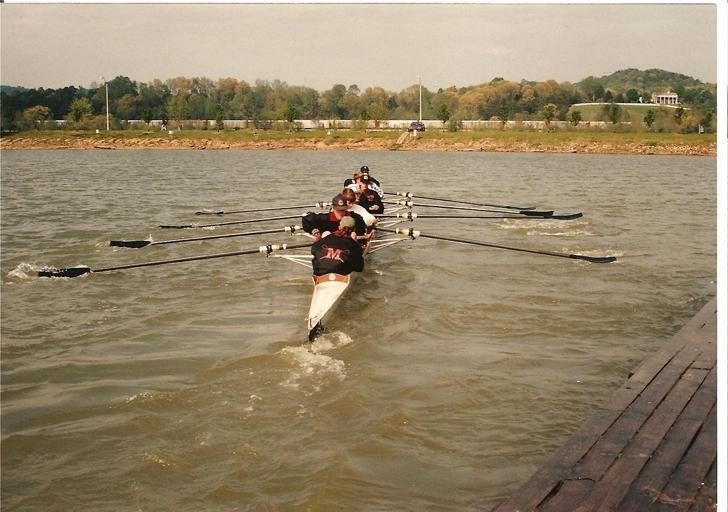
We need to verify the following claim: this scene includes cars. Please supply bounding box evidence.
[407,121,426,132]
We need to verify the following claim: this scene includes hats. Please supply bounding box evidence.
[338,216,356,232]
[332,166,369,211]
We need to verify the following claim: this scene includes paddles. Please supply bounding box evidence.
[368,226,616,262]
[383,192,535,210]
[38,242,315,277]
[373,213,581,219]
[195,202,332,215]
[381,201,553,216]
[109,225,303,248]
[159,213,308,228]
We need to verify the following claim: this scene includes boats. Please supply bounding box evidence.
[304,216,382,342]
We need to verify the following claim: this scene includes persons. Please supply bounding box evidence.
[328,189,379,228]
[159,117,167,131]
[308,214,364,277]
[300,193,368,247]
[342,165,385,215]
